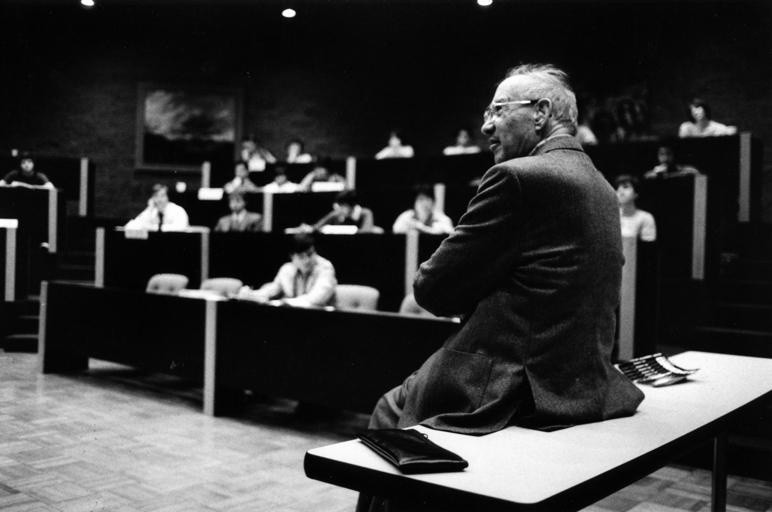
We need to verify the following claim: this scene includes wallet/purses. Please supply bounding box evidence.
[359,428,469,473]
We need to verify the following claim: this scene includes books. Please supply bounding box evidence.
[614,353,698,387]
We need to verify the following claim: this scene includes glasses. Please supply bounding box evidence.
[483,100,538,124]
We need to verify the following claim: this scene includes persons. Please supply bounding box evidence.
[371,61,645,434]
[443,128,482,156]
[127,182,189,226]
[214,192,262,231]
[333,190,374,232]
[224,135,347,192]
[375,131,415,160]
[392,184,454,233]
[576,104,736,241]
[0,151,54,187]
[239,231,337,307]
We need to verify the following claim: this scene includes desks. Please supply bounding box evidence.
[0,122,769,432]
[300,349,772,512]
